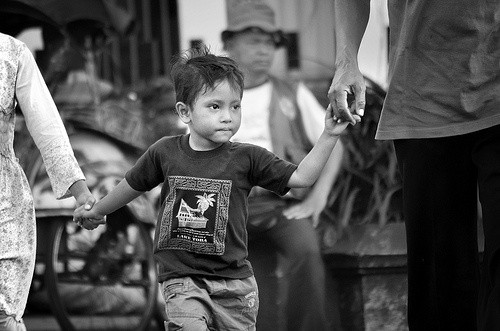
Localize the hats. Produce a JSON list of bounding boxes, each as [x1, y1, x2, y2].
[[221, 1, 282, 42]]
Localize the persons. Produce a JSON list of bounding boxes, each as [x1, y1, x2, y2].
[[73, 45, 355, 331], [326, 0, 500, 331], [0, 33, 107, 330], [185, 0, 346, 331]]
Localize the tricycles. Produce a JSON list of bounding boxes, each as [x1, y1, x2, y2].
[[19, 146, 160, 331]]
